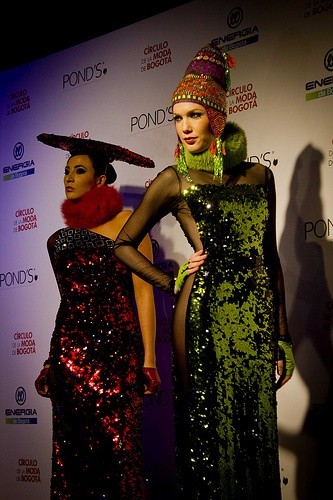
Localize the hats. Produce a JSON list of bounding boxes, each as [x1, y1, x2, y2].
[[37, 131, 155, 169], [171, 42, 234, 138]]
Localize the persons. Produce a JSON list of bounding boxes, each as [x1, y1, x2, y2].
[[109, 75, 295, 500], [34, 131, 162, 500]]
